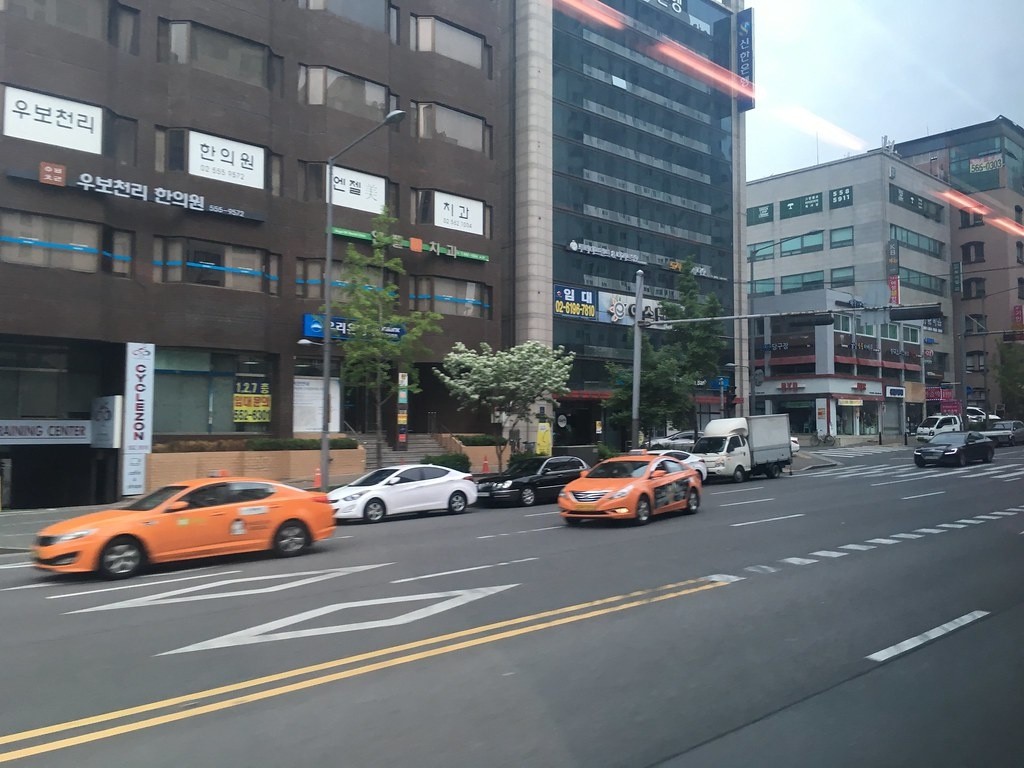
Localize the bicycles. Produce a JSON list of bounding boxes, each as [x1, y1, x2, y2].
[[809, 429, 835, 447]]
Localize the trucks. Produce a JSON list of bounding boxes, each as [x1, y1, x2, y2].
[[915, 415, 964, 443], [691, 414, 793, 484]]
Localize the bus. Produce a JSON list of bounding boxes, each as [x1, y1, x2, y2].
[[631, 448, 708, 484]]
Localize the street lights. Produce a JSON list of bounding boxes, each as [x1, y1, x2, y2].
[[724, 227, 825, 416], [295, 109, 407, 495]]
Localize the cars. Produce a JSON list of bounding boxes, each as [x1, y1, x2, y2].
[[644, 429, 706, 451], [557, 448, 703, 527], [328, 464, 478, 524], [965, 406, 1001, 422], [987, 420, 1024, 447], [30, 469, 337, 581], [477, 455, 591, 508], [913, 430, 994, 468], [791, 437, 800, 453]]
